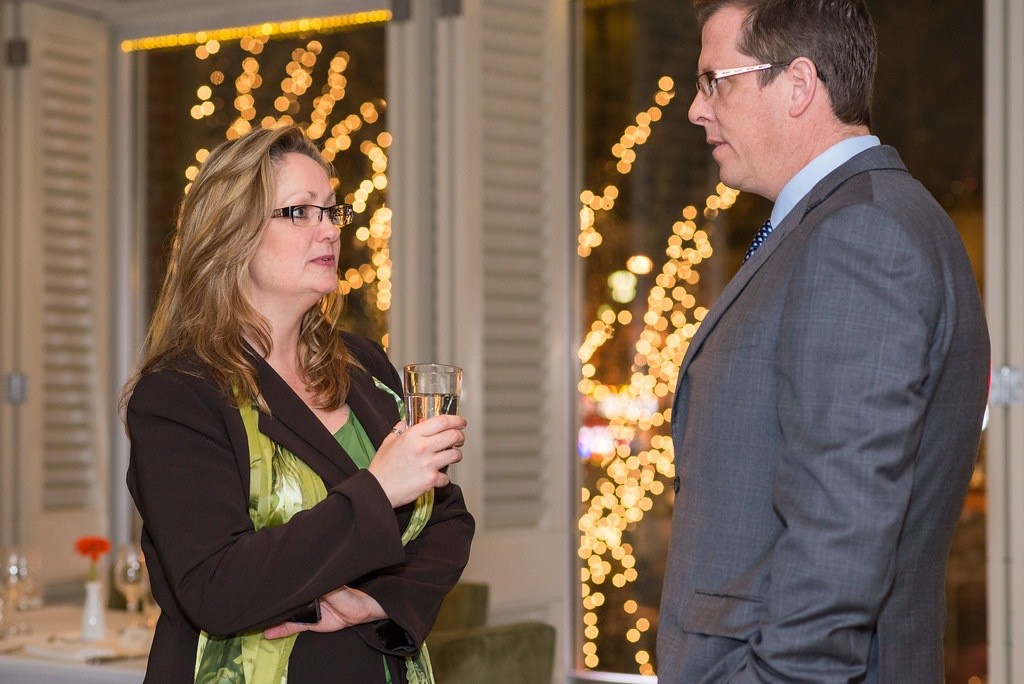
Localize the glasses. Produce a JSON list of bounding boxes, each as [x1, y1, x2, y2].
[[695, 58, 823, 101], [271, 204, 353, 227]]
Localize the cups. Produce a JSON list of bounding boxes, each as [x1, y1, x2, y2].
[[404, 364, 465, 472]]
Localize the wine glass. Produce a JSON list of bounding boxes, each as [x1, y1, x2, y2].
[[3, 544, 41, 637], [113, 542, 154, 648]]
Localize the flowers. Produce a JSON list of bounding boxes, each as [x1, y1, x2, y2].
[[74, 537, 112, 583]]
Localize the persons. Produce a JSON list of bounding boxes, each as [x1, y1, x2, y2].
[[655, 0, 993, 684], [112, 123, 477, 684]]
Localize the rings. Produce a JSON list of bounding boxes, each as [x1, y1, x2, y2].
[[390, 428, 402, 436]]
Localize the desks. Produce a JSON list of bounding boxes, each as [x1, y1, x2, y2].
[[0, 603, 163, 684]]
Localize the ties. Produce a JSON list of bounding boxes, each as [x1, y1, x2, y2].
[[742, 220, 772, 267]]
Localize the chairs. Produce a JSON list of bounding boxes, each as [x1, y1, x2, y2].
[[431, 581, 490, 630], [431, 621, 553, 684]]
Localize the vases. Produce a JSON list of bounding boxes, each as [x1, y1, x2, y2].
[[82, 583, 106, 643]]
